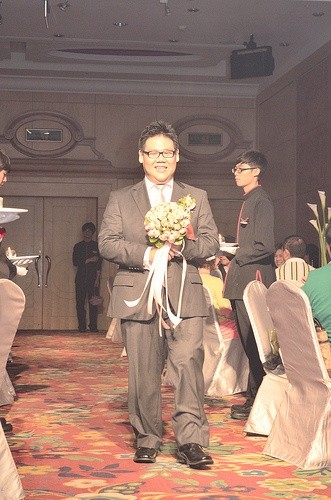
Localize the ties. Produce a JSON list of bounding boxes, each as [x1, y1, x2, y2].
[[153, 184, 167, 204]]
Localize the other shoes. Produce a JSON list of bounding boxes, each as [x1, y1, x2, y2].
[[232, 398, 252, 421]]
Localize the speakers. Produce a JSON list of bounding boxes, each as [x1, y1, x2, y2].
[[230, 45, 274, 79]]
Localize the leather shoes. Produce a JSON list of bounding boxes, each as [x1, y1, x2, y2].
[[132, 443, 160, 465], [172, 441, 214, 468]]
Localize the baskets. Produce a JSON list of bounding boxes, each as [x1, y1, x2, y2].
[[89, 285, 104, 306]]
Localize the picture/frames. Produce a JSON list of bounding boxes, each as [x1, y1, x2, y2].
[[26, 128, 63, 142], [186, 132, 223, 147]]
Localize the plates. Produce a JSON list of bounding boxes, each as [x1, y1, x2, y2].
[[0, 208, 28, 222], [220, 243, 239, 247], [8, 256, 39, 265]]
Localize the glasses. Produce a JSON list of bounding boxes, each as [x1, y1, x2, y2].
[[230, 167, 256, 173], [140, 147, 177, 159]]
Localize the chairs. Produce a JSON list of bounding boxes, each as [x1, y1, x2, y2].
[[106, 280, 117, 340], [242, 280, 288, 438], [0, 422, 26, 500], [0, 279, 25, 406], [201, 286, 250, 398], [262, 281, 331, 471]]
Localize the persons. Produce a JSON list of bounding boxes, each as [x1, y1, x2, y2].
[[0, 150, 28, 431], [98, 120, 220, 466], [196, 234, 331, 348], [72, 222, 102, 332], [220, 151, 275, 420]]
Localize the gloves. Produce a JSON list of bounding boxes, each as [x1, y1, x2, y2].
[[15, 263, 30, 275]]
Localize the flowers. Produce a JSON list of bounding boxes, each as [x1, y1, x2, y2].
[[89, 288, 104, 306], [124, 194, 197, 338]]
